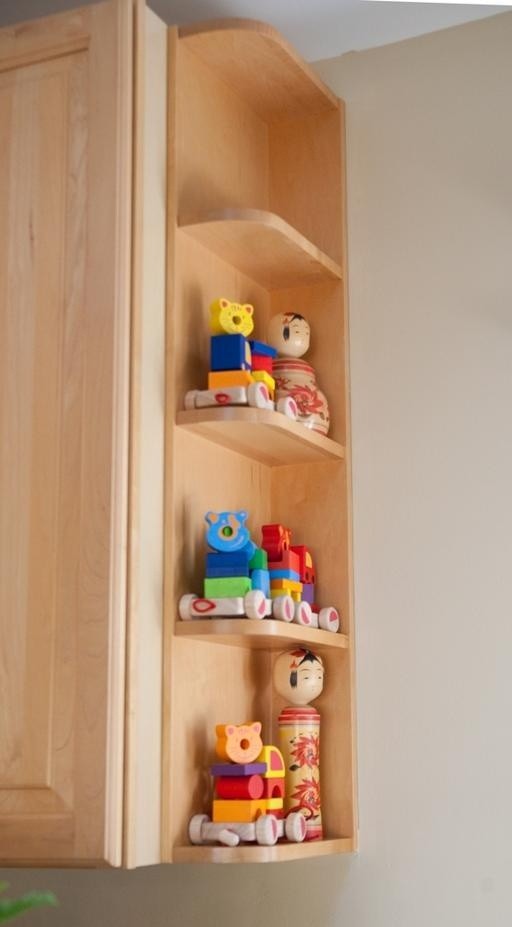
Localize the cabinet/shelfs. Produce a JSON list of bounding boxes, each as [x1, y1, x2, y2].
[[1, 2, 135, 869], [160, 16, 360, 864]]
